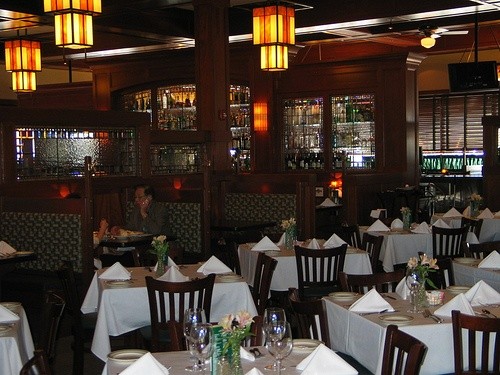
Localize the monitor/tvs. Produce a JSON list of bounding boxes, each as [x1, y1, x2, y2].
[[448, 60, 498, 95]]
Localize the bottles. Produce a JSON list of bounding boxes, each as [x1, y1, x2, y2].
[[162, 93, 197, 130], [286, 152, 343, 170], [232, 88, 250, 150]]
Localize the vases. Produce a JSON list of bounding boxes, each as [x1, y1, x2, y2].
[[157, 255, 166, 277], [231, 343, 243, 375]]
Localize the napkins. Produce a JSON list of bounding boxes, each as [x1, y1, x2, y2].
[[466, 280, 500, 306], [197, 255, 232, 275], [252, 236, 279, 253], [349, 289, 394, 313], [98, 262, 131, 281], [434, 293, 475, 317], [156, 266, 188, 282], [297, 344, 358, 375], [119, 352, 169, 375], [324, 233, 351, 249]]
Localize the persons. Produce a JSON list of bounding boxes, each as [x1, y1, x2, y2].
[[124, 183, 175, 270], [64, 192, 129, 270]]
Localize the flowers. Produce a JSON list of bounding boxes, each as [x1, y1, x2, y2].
[[408, 251, 440, 303], [279, 218, 296, 247], [223, 311, 255, 375], [151, 235, 169, 272]]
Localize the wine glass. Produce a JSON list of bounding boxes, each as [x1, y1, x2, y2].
[[263, 308, 292, 375], [183, 308, 214, 375]]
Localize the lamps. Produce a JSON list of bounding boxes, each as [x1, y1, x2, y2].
[[420, 27, 436, 48], [250, 2, 297, 71], [4, 0, 102, 91]]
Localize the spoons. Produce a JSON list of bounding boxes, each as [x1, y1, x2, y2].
[[422, 310, 443, 323]]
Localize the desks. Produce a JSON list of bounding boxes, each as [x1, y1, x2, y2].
[[0, 217, 500, 375], [235, 241, 373, 300], [350, 221, 482, 277], [81, 261, 258, 364], [102, 339, 358, 375], [432, 207, 500, 243], [322, 287, 500, 375], [0, 301, 39, 375], [451, 255, 500, 291]]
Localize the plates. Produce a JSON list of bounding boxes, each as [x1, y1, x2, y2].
[[104, 280, 134, 286], [216, 274, 246, 280], [114, 236, 128, 239], [0, 326, 8, 333], [330, 292, 357, 299], [446, 285, 473, 291], [287, 339, 325, 350], [0, 302, 20, 309], [454, 258, 477, 263], [13, 252, 33, 255], [107, 349, 150, 362], [378, 315, 414, 323]]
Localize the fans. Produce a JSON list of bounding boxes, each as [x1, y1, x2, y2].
[[394, 26, 469, 49]]
